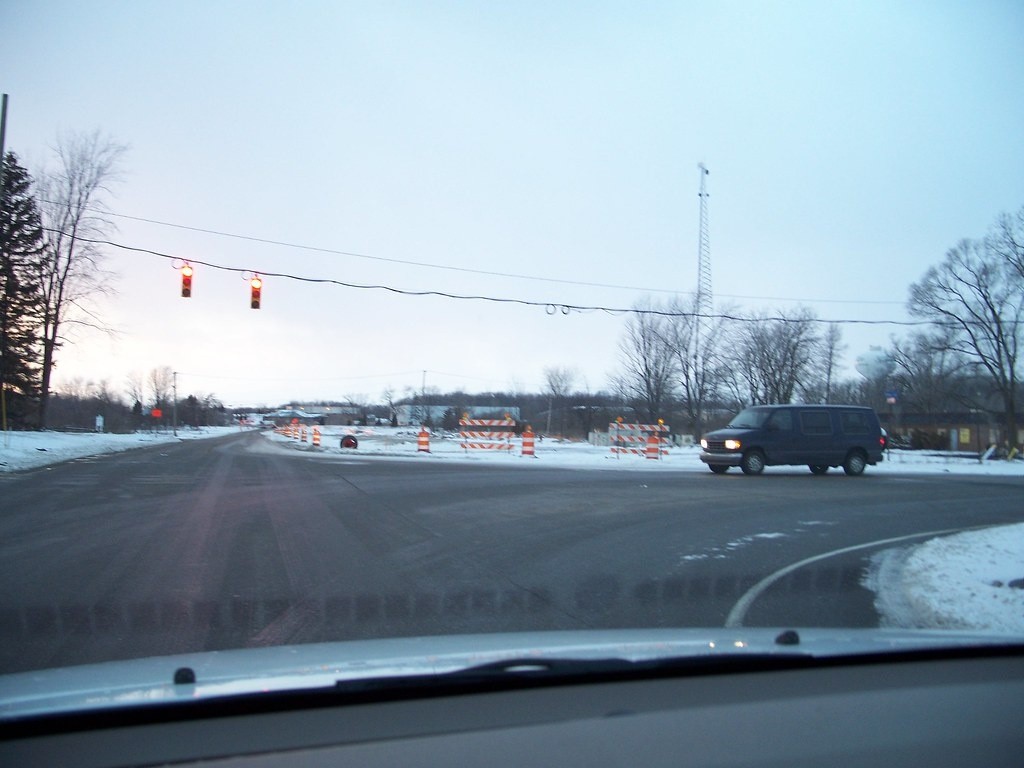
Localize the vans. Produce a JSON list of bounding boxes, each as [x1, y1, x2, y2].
[[699, 404, 888, 476]]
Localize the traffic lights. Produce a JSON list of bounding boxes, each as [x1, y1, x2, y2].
[[251, 277, 261, 309], [182, 266, 192, 297]]
